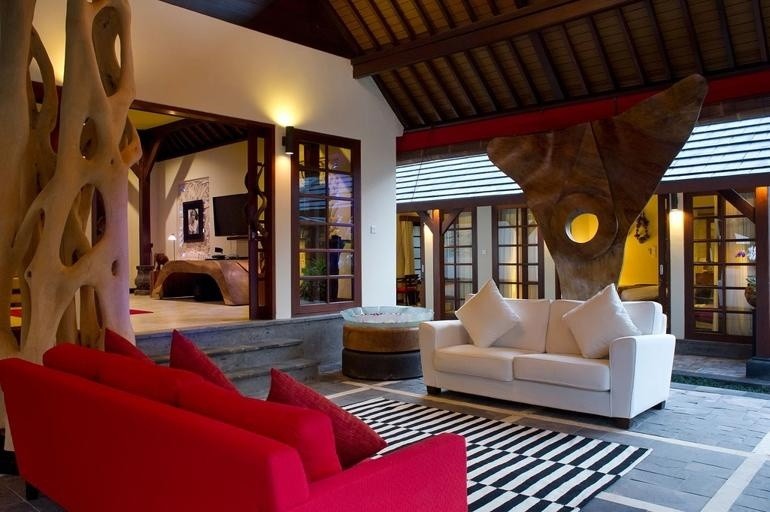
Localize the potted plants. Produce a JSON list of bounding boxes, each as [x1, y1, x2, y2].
[[745, 274, 756, 310], [299, 254, 328, 304]]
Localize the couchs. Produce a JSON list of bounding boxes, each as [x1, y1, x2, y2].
[[417, 291, 678, 430], [0, 341, 470, 512]]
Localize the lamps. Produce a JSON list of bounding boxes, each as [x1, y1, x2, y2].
[[281, 125, 296, 157], [167, 233, 178, 262], [670, 193, 679, 210]]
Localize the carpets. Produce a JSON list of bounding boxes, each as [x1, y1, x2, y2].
[[337, 395, 655, 512], [130, 307, 154, 317]]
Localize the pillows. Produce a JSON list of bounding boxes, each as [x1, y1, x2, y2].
[[167, 329, 239, 395], [264, 367, 387, 466], [454, 278, 522, 348], [562, 281, 641, 359], [103, 327, 152, 361]]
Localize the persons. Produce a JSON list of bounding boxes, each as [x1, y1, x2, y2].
[[187, 209, 199, 234]]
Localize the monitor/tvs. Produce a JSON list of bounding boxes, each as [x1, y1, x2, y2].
[[213, 193, 249, 236]]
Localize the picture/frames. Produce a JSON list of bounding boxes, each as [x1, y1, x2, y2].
[[182, 199, 205, 243]]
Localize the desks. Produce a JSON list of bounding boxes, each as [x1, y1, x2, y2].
[[153, 259, 249, 307]]
